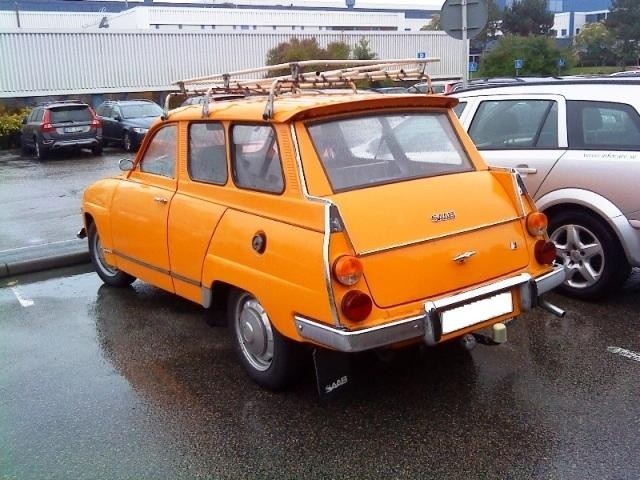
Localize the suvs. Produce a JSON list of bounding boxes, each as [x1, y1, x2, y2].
[[19, 101, 105, 160]]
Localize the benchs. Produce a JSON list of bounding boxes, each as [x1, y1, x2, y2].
[[328, 161, 393, 190]]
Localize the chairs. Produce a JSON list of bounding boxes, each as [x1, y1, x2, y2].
[[581, 106, 603, 144], [197, 143, 244, 182], [483, 111, 520, 148]]
